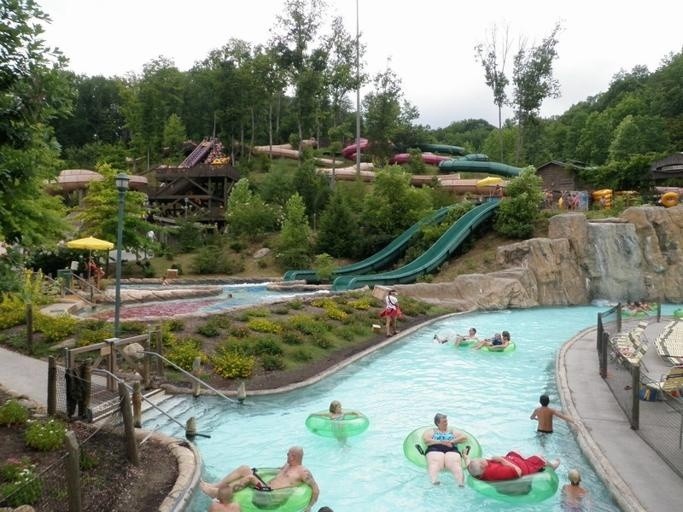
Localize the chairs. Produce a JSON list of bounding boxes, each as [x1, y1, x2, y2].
[[608, 315, 683, 401]]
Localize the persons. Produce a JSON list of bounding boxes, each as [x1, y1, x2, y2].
[[600, 195, 606, 209], [563, 470, 587, 512], [198, 446, 318, 512], [421, 413, 464, 487], [468, 450, 560, 482], [530, 394, 575, 432], [313, 401, 359, 419], [82, 253, 105, 283], [319, 507, 334, 512], [379, 289, 400, 336], [432, 328, 511, 351], [207, 487, 240, 512], [622, 297, 654, 318]]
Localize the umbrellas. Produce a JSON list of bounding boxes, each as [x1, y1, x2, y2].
[[479, 176, 503, 195], [65, 234, 114, 276]]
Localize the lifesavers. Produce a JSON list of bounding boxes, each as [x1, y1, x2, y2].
[[674, 309, 683, 319], [403, 426, 483, 470], [481, 340, 515, 353], [618, 303, 657, 317], [229, 468, 312, 512], [557, 188, 680, 211], [306, 409, 368, 437], [450, 339, 478, 348], [465, 465, 559, 503]]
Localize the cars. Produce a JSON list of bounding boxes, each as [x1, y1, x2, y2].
[[20, 247, 88, 281]]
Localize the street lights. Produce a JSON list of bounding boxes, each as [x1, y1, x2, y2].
[[114, 172, 129, 365]]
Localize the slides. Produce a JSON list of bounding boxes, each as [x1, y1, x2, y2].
[[283, 206, 448, 284], [331, 200, 501, 291]]
